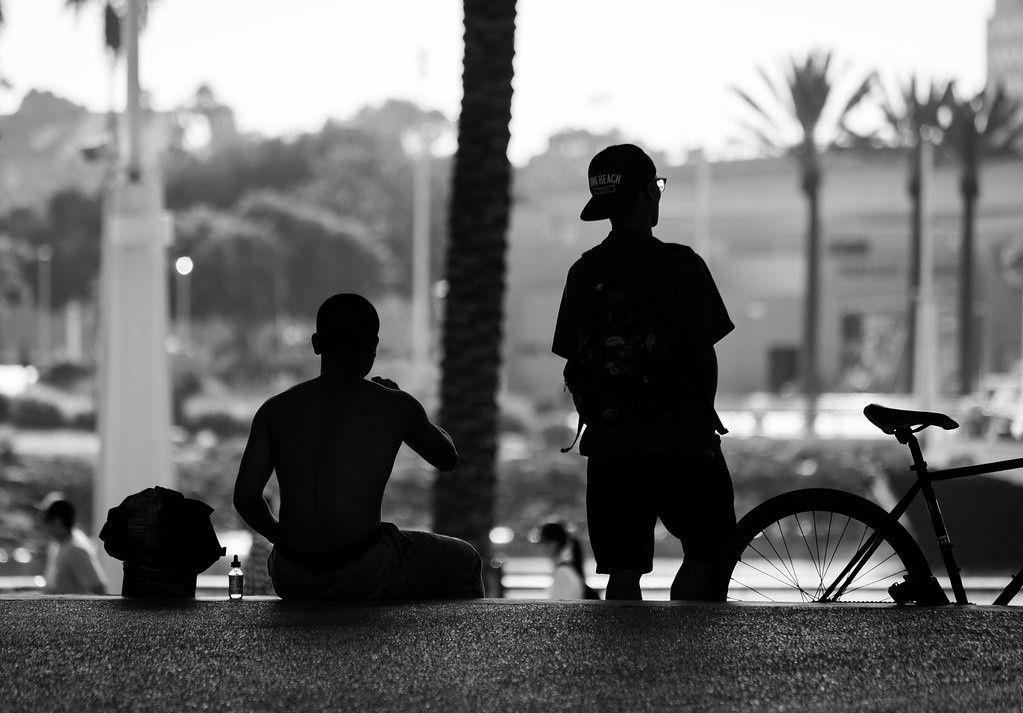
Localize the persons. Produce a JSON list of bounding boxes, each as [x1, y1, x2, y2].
[[39, 499, 104, 595], [549, 142, 737, 602], [539, 523, 590, 601], [240, 496, 278, 596], [234, 293, 488, 604]]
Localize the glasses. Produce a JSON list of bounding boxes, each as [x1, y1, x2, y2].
[[656, 178, 667, 193]]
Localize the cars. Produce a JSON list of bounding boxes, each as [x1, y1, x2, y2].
[[0, 348, 588, 584]]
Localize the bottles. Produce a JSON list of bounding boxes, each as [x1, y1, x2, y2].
[[228, 555, 244, 600]]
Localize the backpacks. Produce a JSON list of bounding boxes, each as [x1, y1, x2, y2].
[[559, 562, 605, 600]]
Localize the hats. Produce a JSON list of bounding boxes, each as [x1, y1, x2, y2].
[[580, 144, 657, 222], [30, 500, 76, 520], [527, 523, 574, 543]]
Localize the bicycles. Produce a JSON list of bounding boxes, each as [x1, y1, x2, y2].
[[721, 403, 1023, 606]]
